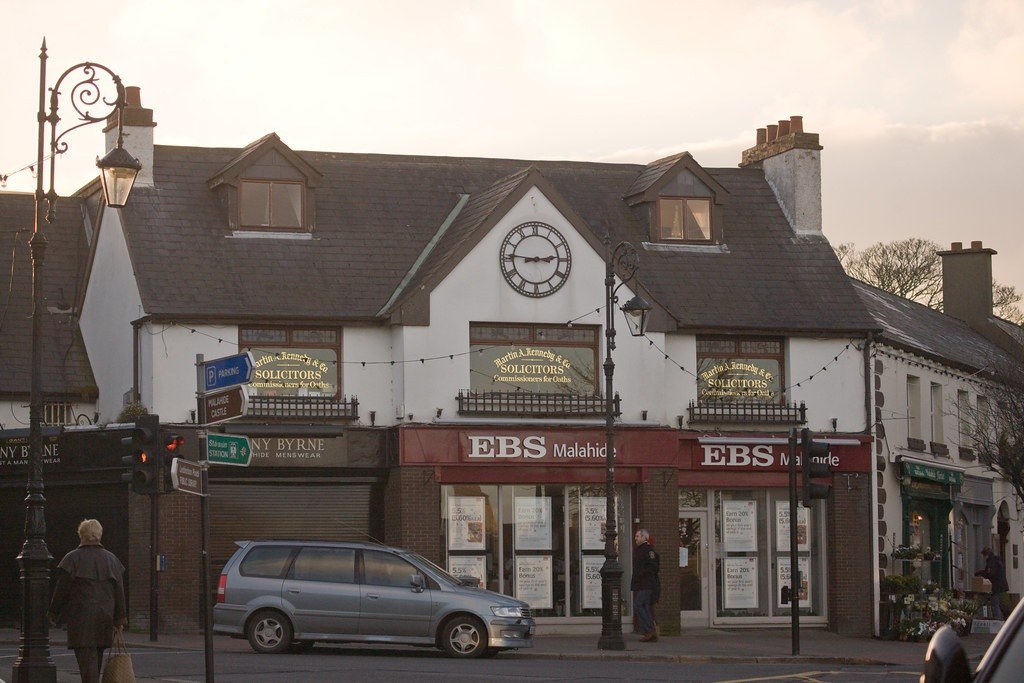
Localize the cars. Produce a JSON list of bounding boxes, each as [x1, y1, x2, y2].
[[919, 595, 1024, 683]]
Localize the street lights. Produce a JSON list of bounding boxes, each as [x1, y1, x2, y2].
[[592, 225, 654, 649], [9, 34, 140, 683]]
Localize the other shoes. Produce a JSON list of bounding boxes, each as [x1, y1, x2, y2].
[[640, 630, 658, 643], [984, 616, 1003, 621]]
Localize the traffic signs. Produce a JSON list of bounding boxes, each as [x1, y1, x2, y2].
[[206, 433, 252, 466], [205, 382, 249, 425], [171, 458, 204, 496], [202, 351, 256, 393]]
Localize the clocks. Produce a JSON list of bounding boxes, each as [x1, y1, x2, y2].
[[500, 222, 573, 298]]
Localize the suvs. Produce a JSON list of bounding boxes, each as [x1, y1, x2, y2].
[[208, 537, 537, 660]]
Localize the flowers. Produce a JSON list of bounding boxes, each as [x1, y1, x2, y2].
[[898, 619, 912, 635], [907, 545, 920, 554], [895, 547, 908, 556], [925, 547, 934, 555]]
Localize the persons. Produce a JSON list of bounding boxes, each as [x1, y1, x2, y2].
[[49, 519, 129, 683], [975, 547, 1008, 620], [630, 531, 660, 643]]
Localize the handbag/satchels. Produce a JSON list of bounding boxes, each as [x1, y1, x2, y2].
[[102, 630, 136, 683]]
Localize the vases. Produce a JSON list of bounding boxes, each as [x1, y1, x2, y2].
[[896, 554, 905, 559], [924, 553, 934, 560], [906, 554, 918, 560], [899, 633, 908, 641]]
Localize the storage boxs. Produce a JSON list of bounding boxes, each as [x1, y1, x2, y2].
[[972, 577, 992, 592], [971, 620, 1004, 634]]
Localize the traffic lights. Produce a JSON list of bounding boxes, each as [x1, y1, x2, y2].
[[159, 429, 187, 491], [130, 414, 160, 497], [121, 437, 134, 487], [801, 425, 836, 507]]
